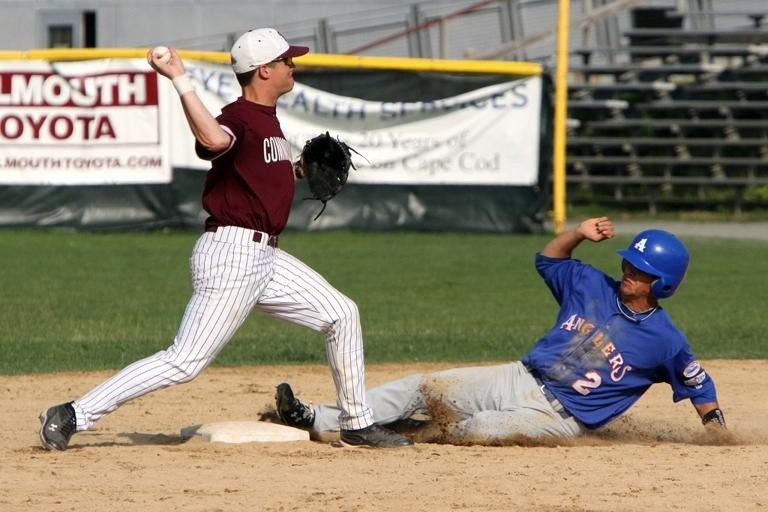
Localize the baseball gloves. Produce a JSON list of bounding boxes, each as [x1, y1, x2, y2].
[[298, 131, 371, 220]]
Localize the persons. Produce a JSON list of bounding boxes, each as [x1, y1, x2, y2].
[[38, 26, 416, 449], [275, 217, 742, 447]]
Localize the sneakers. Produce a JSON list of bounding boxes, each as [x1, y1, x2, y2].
[[40, 401, 76, 451], [340, 423, 413, 447], [275, 383, 314, 425]]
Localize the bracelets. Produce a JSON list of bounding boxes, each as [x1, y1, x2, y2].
[[171, 73, 195, 98], [701, 407, 727, 431]]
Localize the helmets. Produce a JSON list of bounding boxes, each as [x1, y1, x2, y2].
[[617, 229, 689, 298]]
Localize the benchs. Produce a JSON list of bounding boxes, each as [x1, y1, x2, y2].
[[546, 28, 768, 216]]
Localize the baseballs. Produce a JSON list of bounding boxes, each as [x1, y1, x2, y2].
[[153, 47, 170, 64]]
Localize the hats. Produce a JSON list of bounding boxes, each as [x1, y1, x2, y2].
[[231, 27, 309, 75]]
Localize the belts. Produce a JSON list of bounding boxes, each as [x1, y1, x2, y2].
[[206, 225, 278, 247], [522, 361, 572, 419]]
